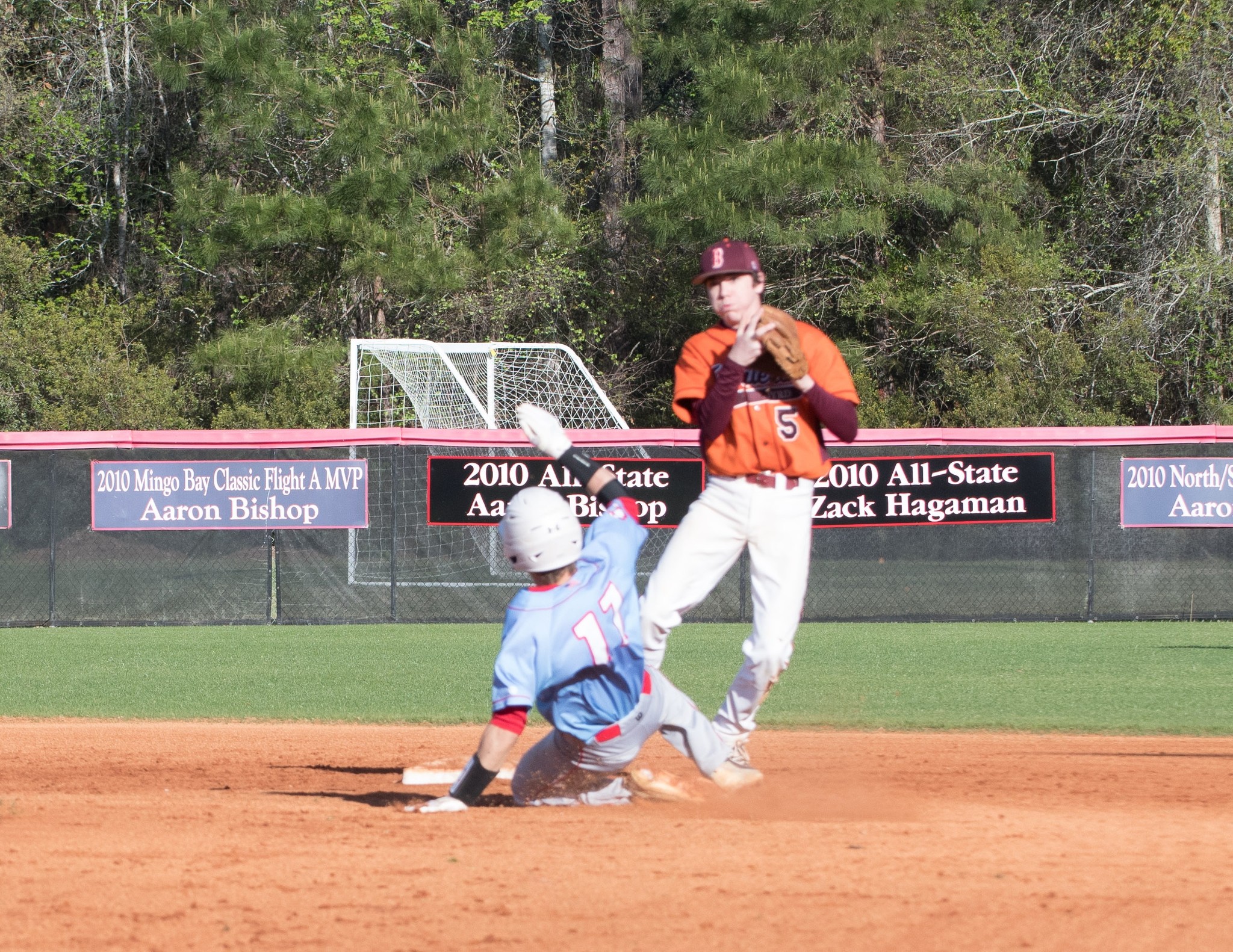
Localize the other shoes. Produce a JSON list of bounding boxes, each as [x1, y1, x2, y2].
[[712, 761, 762, 791], [730, 741, 749, 766], [623, 768, 705, 804]]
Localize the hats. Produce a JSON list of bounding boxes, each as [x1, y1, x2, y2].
[[692, 238, 761, 285]]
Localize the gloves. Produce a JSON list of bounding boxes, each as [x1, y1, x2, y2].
[[404, 796, 468, 813], [516, 403, 573, 460]]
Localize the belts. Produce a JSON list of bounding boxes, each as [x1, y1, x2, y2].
[[562, 671, 651, 746], [746, 473, 799, 490]]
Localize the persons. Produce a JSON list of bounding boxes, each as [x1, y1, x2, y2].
[[639, 237, 860, 767], [403, 403, 765, 813]]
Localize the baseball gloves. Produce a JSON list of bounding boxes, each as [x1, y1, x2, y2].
[[746, 305, 809, 381]]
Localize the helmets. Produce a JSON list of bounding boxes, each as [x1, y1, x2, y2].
[[501, 486, 583, 572]]
